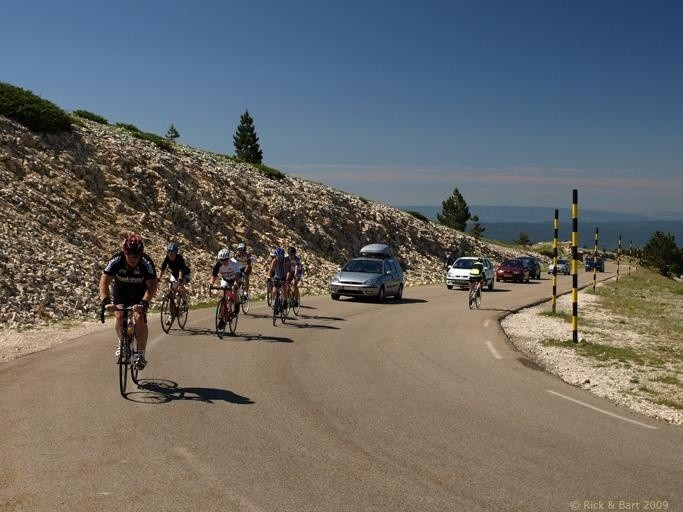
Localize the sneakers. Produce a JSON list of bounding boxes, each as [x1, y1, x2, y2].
[[134, 355, 146, 370], [116, 344, 128, 357]]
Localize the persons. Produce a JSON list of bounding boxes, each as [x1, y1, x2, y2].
[[157, 242, 302, 329], [99, 236, 157, 370], [469, 259, 487, 305]]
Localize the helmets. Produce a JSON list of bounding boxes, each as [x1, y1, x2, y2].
[[271, 246, 296, 258], [166, 242, 178, 253], [122, 236, 143, 255], [237, 242, 246, 250], [218, 248, 230, 261]]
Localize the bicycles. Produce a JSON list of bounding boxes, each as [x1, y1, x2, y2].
[[99, 302, 152, 396], [157, 277, 190, 335], [230, 277, 250, 316], [204, 284, 241, 340], [468, 278, 482, 309], [263, 275, 300, 327]]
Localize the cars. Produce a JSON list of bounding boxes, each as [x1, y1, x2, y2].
[[495, 259, 529, 283], [517, 256, 542, 282], [546, 258, 570, 275], [328, 244, 403, 301], [444, 256, 494, 291], [584, 257, 605, 273]]
